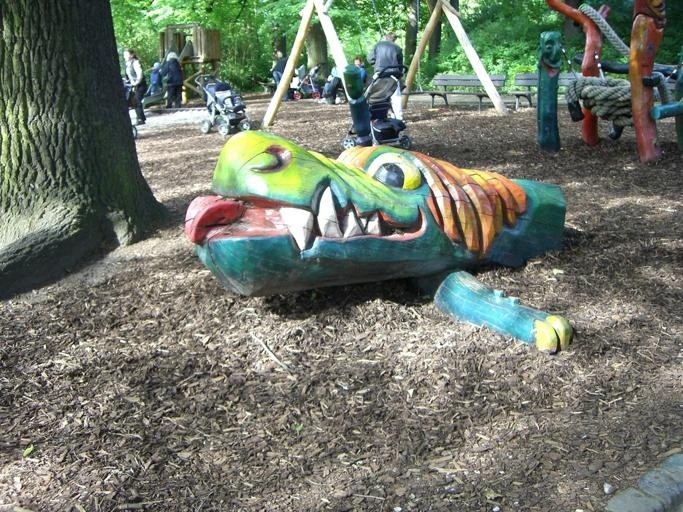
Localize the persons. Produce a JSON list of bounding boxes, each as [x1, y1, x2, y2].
[[321, 56, 367, 99], [272, 56, 296, 103], [318, 66, 341, 104], [123, 48, 148, 127], [366, 32, 406, 124], [268, 50, 284, 74], [158, 50, 182, 108], [149, 61, 161, 96]]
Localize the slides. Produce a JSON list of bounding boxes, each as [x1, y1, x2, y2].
[[141, 40, 194, 108]]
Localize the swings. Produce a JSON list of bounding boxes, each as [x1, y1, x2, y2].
[[350, 0, 424, 95]]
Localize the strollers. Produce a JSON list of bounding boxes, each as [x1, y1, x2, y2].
[[339, 64, 413, 150], [293, 61, 328, 100], [194, 73, 252, 136]]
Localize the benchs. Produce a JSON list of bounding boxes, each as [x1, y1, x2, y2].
[[426, 73, 580, 113]]
